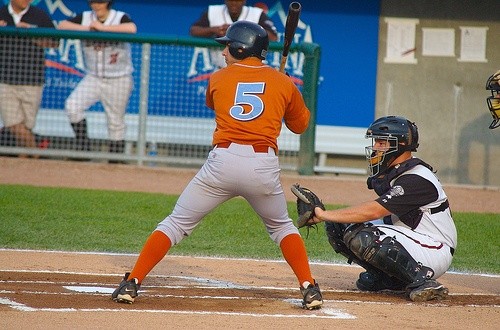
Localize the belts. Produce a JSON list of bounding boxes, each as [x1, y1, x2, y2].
[[217, 141, 268, 152], [450, 248, 454, 256]]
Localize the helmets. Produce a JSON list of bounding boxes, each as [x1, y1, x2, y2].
[[486, 72, 500, 129], [365, 116, 419, 177], [89, 0, 112, 6], [215, 21, 269, 60]]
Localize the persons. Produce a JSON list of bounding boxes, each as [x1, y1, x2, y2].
[[0, 0, 61, 159], [292, 116, 458, 301], [112, 21, 323, 310], [190, 0, 278, 75], [57, 0, 137, 164], [486, 71, 500, 129]]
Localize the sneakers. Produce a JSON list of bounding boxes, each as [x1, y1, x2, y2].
[[356, 272, 406, 292], [300, 279, 323, 310], [112, 272, 140, 305], [410, 279, 449, 301]]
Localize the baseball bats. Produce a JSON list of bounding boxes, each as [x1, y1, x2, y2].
[[278, 1, 302, 71]]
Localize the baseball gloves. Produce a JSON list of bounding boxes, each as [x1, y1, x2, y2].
[[288, 184, 326, 230]]
